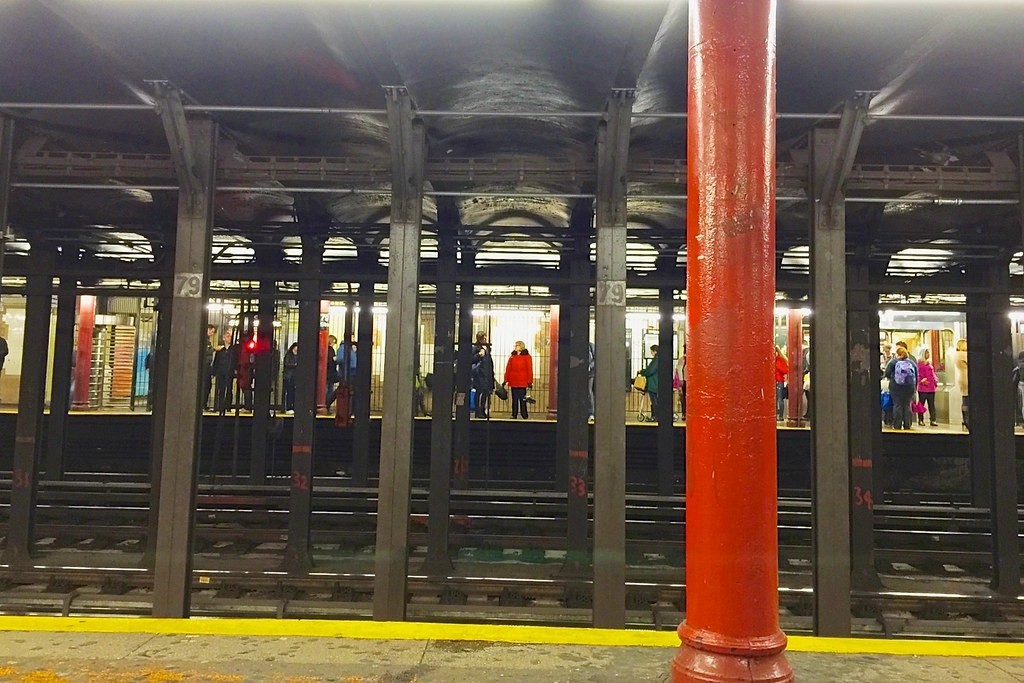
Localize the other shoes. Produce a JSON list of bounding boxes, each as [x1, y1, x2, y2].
[[893, 426, 901, 429], [918, 420, 926, 426], [903, 424, 910, 429], [509, 415, 517, 419], [522, 415, 528, 419], [145, 399, 338, 417], [681, 415, 686, 421], [588, 415, 595, 422], [424, 413, 432, 417], [930, 421, 938, 426]]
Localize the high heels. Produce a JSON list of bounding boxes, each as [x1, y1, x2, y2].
[[475, 410, 485, 419], [962, 421, 968, 432], [481, 410, 492, 418]]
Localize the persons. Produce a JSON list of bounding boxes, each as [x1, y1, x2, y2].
[[677, 343, 687, 421], [1013, 350, 1024, 424], [775, 344, 788, 420], [203, 324, 298, 415], [0, 337, 8, 372], [416, 330, 534, 421], [802, 340, 812, 421], [879, 338, 969, 432], [326, 332, 358, 424], [637, 344, 658, 421], [588, 342, 595, 424]]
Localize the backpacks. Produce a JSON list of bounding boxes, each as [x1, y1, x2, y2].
[[892, 359, 916, 386]]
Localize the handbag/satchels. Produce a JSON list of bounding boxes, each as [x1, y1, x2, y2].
[[911, 400, 927, 413], [633, 371, 648, 393], [673, 371, 680, 388], [524, 387, 536, 405], [495, 382, 510, 400]]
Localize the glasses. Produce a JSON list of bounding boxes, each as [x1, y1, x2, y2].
[[514, 345, 522, 347]]
